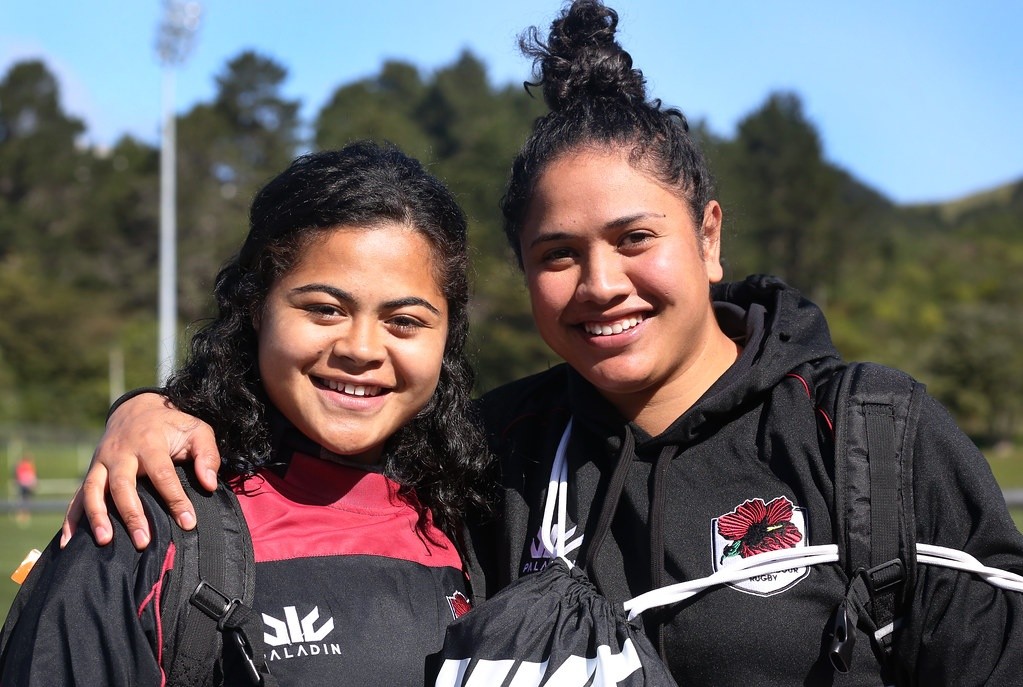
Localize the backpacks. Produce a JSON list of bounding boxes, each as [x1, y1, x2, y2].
[[432, 412, 1023, 687]]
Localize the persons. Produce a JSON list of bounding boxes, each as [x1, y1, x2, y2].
[[0, 0, 1023, 687]]
